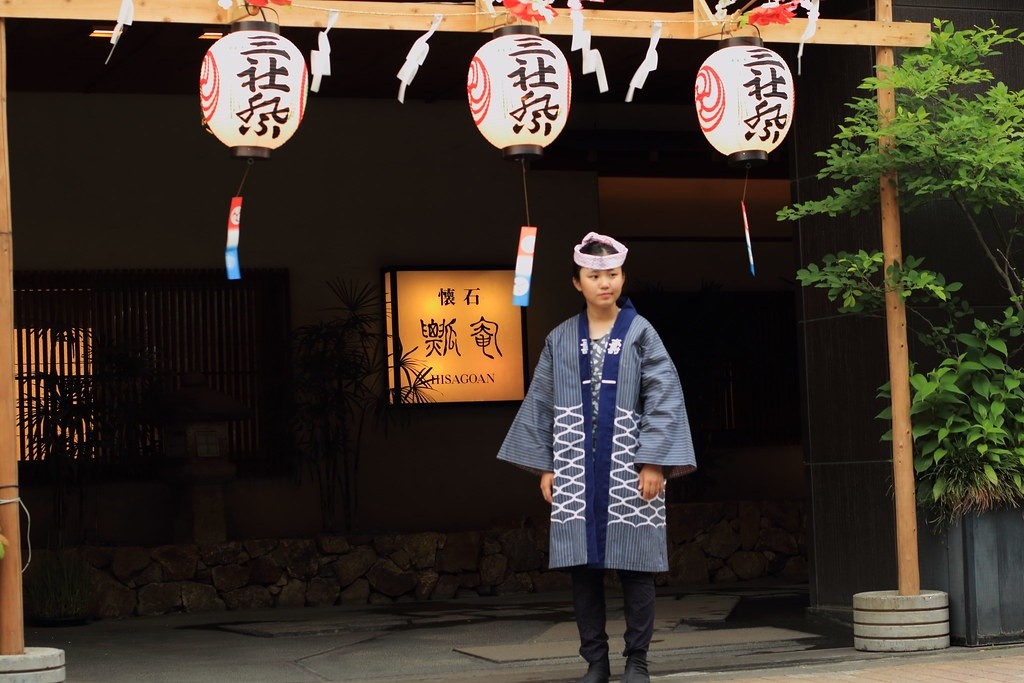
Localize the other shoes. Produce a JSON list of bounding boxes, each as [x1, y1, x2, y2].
[[578, 659, 611, 683], [619, 649, 650, 683]]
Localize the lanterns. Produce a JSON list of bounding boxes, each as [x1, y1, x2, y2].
[[198, 20, 310, 159], [466, 23, 573, 156], [693, 36, 798, 163]]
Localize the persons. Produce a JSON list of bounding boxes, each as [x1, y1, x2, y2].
[[495, 231, 697, 683]]
